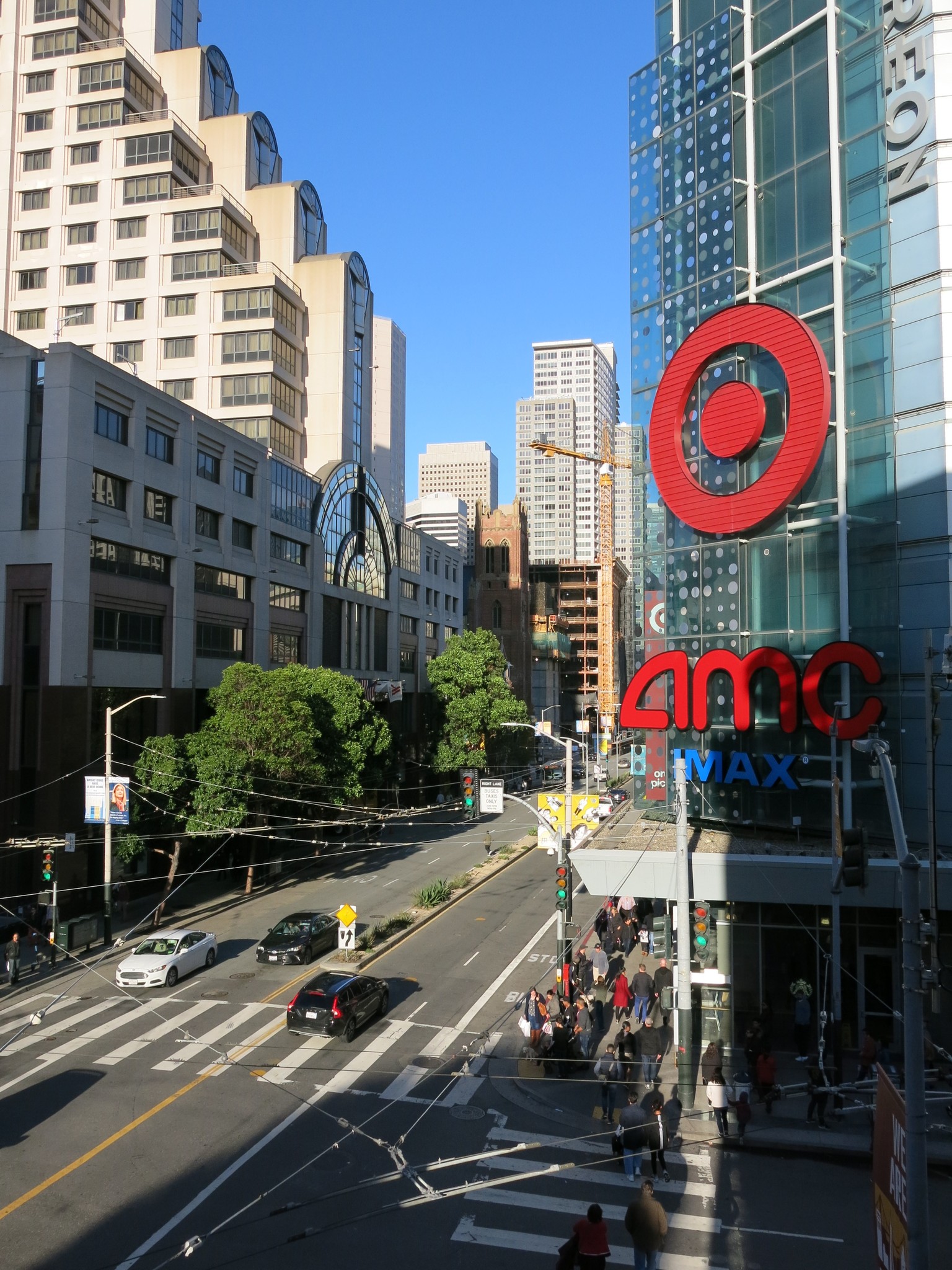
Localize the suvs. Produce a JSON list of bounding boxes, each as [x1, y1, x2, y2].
[[598, 788, 630, 819], [572, 763, 586, 778], [286, 971, 389, 1044]]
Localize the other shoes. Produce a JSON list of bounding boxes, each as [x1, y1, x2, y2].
[[718, 1131, 744, 1144], [608, 1119, 615, 1124], [645, 1084, 651, 1089], [596, 1028, 605, 1032], [601, 1114, 607, 1120], [642, 951, 648, 956], [616, 1016, 646, 1026], [651, 1076, 657, 1083], [556, 1074, 567, 1078], [625, 954, 628, 958], [623, 1083, 629, 1090]]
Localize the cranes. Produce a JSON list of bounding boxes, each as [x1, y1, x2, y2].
[[531, 422, 630, 730]]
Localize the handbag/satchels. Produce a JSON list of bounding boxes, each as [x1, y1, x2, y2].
[[609, 978, 617, 992], [558, 1231, 579, 1264], [518, 1016, 531, 1037], [537, 993, 547, 1016], [615, 940, 625, 952], [598, 1074, 608, 1085], [542, 1019, 553, 1037]]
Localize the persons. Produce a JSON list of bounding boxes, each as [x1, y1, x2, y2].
[[520, 777, 532, 796], [3, 932, 21, 986], [446, 792, 453, 809], [619, 1091, 671, 1182], [112, 876, 130, 922], [436, 791, 444, 810], [283, 922, 299, 934], [854, 1027, 875, 1089], [922, 1019, 934, 1091], [701, 1018, 777, 1146], [484, 831, 493, 856], [594, 896, 654, 958], [572, 1204, 610, 1270], [808, 1050, 832, 1130], [525, 943, 673, 1125], [625, 1180, 668, 1270], [21, 899, 60, 948]]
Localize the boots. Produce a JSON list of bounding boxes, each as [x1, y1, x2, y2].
[[533, 1029, 540, 1047], [529, 1029, 536, 1046]]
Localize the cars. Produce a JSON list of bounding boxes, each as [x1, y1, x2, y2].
[[588, 753, 597, 761], [115, 929, 218, 988], [618, 758, 630, 768], [593, 768, 610, 782], [255, 912, 339, 965]]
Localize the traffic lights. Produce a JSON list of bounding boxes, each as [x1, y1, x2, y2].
[[661, 990, 673, 1009], [554, 864, 567, 910], [694, 902, 710, 962], [459, 767, 482, 821], [38, 892, 51, 905], [41, 849, 54, 882], [842, 829, 865, 888], [606, 754, 609, 762], [651, 915, 672, 959]]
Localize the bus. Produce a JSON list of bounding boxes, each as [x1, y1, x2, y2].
[[541, 759, 565, 787]]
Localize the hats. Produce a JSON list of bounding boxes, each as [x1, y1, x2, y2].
[[560, 997, 570, 1002], [642, 924, 648, 930], [580, 945, 588, 949], [594, 943, 601, 948]]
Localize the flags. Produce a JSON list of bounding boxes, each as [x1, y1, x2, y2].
[[374, 680, 391, 693], [387, 681, 402, 703], [360, 679, 376, 701]]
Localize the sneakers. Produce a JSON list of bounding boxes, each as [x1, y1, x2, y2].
[[627, 1170, 670, 1183]]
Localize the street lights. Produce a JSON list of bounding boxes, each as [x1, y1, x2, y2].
[[541, 705, 560, 764], [852, 737, 925, 1270], [830, 702, 849, 1110], [616, 734, 623, 782], [581, 702, 597, 763], [104, 694, 166, 945], [500, 722, 573, 1007]]
